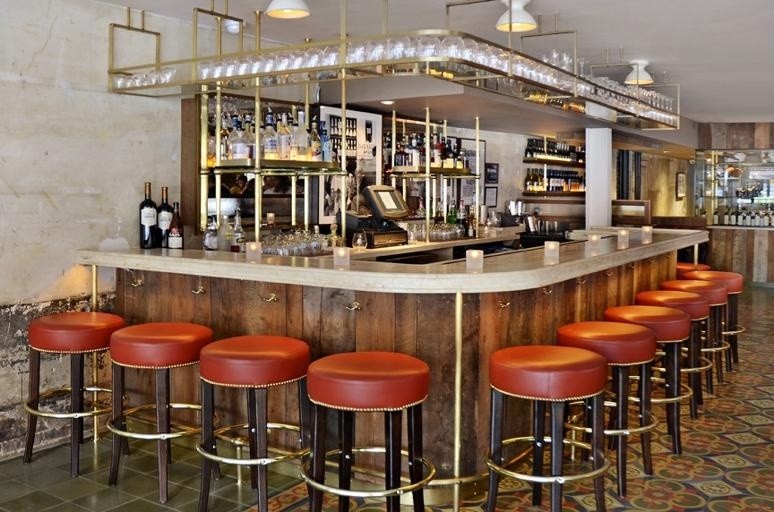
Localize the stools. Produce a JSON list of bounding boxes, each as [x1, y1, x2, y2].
[[193, 334, 312, 512], [108, 322, 223, 505], [487, 346, 605, 512], [605, 305, 692, 456], [641, 264, 743, 420], [18, 311, 129, 479], [297, 349, 437, 512], [558, 319, 653, 499]]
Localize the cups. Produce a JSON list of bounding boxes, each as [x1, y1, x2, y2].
[[406, 223, 464, 242], [331, 246, 349, 267], [246, 242, 260, 260], [352, 233, 367, 250], [588, 232, 602, 249], [489, 211, 501, 229], [617, 241, 628, 250], [465, 249, 483, 269], [553, 219, 559, 233], [641, 237, 652, 244], [112, 35, 673, 125], [544, 221, 550, 233], [258, 224, 342, 257], [544, 241, 559, 257], [617, 230, 629, 241], [641, 226, 653, 237], [266, 213, 274, 224]]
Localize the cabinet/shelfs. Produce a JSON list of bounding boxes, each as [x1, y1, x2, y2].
[[196, 88, 348, 249], [701, 162, 773, 201], [383, 113, 461, 226], [522, 156, 586, 198]]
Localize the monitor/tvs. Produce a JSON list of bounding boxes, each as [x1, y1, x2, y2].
[[361, 185, 410, 228]]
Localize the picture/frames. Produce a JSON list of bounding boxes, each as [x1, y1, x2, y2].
[[484, 187, 497, 207], [676, 172, 687, 197], [484, 163, 498, 184]]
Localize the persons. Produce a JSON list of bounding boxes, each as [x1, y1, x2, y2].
[[324, 146, 376, 215], [208, 175, 304, 198]]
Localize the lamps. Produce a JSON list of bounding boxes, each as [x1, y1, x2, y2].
[[623, 59, 653, 87], [494, 0, 537, 34], [265, 0, 312, 20]]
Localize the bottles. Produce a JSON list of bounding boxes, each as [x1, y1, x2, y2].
[[138, 181, 158, 248], [203, 109, 332, 164], [383, 129, 468, 169], [156, 185, 174, 247], [699, 207, 774, 228], [167, 202, 184, 249], [525, 138, 584, 162], [523, 168, 582, 191], [202, 208, 246, 252], [434, 199, 487, 236]]
